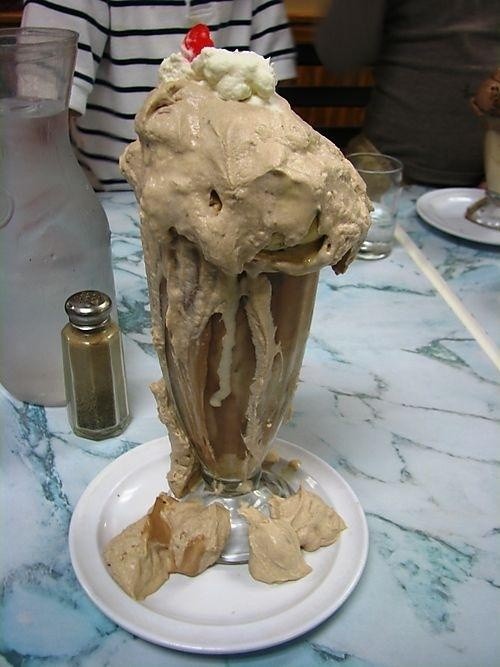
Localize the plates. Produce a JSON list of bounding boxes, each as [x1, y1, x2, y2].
[[67, 433, 370, 655], [416, 188, 500, 246]]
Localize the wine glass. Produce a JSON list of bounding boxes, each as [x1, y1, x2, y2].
[[146, 219, 335, 562]]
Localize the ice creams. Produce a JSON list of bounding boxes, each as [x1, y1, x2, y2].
[[103, 24, 373, 601], [465, 65, 500, 230]]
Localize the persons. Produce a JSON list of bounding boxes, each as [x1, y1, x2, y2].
[[312, 1, 500, 204], [14, 0, 299, 192]]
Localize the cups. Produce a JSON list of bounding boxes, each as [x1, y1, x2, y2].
[[344, 152, 403, 261]]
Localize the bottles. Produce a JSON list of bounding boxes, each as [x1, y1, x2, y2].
[[1, 24, 118, 409], [466, 115, 499, 229]]
